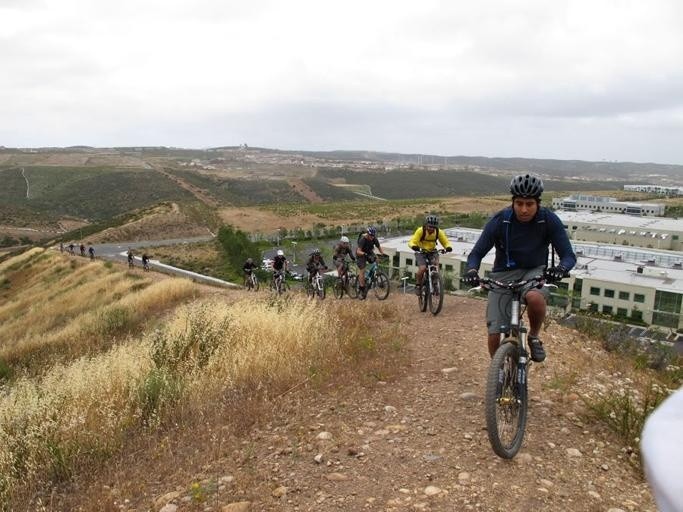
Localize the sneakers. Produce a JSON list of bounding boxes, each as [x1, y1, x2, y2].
[[496, 368, 505, 398], [415, 283, 422, 295], [527, 334, 546, 363]]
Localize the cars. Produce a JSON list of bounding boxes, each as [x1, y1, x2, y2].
[[397, 283, 409, 288], [260, 257, 306, 284]]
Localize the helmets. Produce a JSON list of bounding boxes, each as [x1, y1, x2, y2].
[[425, 215, 439, 227], [277, 249, 283, 256], [339, 236, 349, 243], [508, 173, 544, 200], [313, 248, 320, 256], [366, 226, 376, 236]]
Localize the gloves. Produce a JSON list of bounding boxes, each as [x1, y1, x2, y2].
[[544, 263, 568, 283], [411, 246, 420, 251], [445, 247, 452, 252], [461, 271, 480, 287]]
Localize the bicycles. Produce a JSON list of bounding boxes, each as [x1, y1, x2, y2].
[[330, 256, 363, 299], [68, 249, 151, 276], [454, 268, 570, 460], [353, 252, 393, 300], [305, 265, 327, 301], [413, 246, 452, 314], [241, 266, 261, 293], [269, 272, 291, 299]]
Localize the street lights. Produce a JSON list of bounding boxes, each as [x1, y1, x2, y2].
[[292, 241, 297, 264], [276, 228, 280, 247], [401, 275, 408, 295]]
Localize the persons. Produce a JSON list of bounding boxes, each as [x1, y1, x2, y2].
[[408, 215, 452, 296], [355, 227, 383, 298], [242, 258, 258, 288], [332, 236, 355, 293], [59, 240, 150, 273], [306, 249, 328, 285], [272, 250, 289, 283], [460, 174, 577, 395]]
